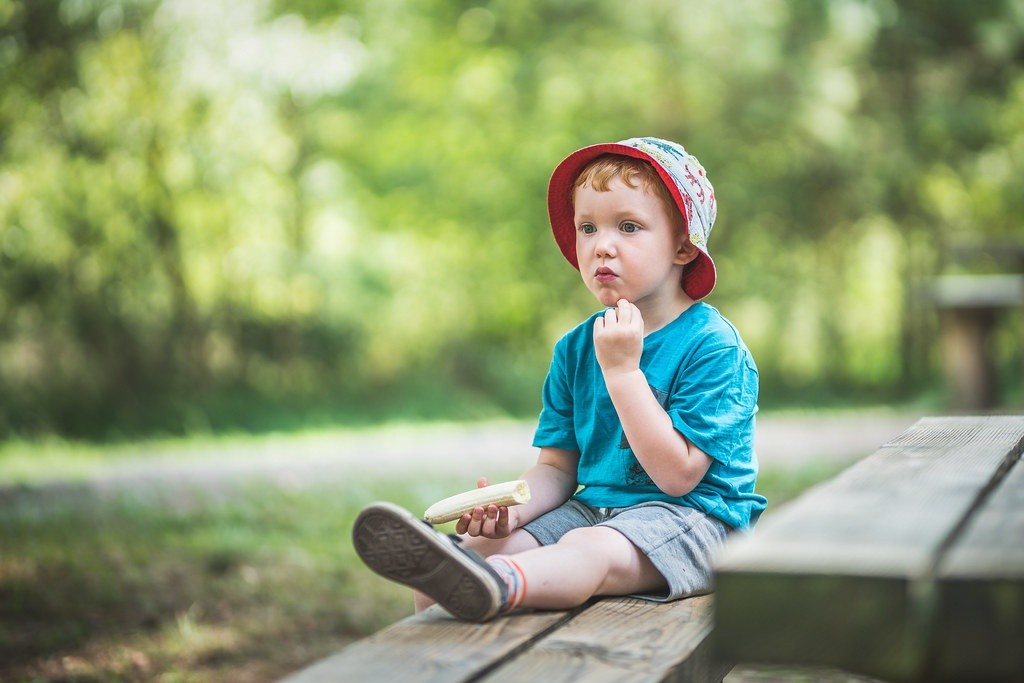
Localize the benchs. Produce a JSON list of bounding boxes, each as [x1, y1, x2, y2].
[[280, 592, 737, 683]]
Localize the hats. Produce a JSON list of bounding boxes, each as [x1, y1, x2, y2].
[[548, 136, 717, 301]]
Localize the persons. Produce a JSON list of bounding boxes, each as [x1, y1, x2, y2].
[[352, 138, 769, 622]]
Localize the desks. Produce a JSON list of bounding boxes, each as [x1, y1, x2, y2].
[[709, 416, 1024, 683], [932, 275, 1024, 414]]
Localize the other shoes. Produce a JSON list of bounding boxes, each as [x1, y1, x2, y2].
[[351, 501, 510, 623]]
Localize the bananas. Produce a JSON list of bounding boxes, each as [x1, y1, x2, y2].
[[424, 480, 531, 523]]
